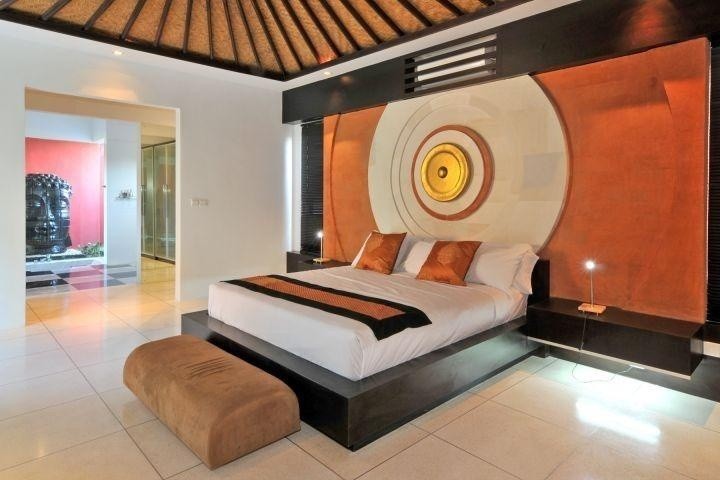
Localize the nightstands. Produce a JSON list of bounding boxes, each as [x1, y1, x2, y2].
[[297, 260, 351, 272], [528, 298, 704, 381]]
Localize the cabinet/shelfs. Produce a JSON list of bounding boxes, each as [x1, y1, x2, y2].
[[141, 147, 154, 256], [153, 142, 175, 263]]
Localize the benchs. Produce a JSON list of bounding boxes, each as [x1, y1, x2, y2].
[[123, 335, 301, 471]]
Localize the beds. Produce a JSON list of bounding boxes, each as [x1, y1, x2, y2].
[[182, 258, 550, 452]]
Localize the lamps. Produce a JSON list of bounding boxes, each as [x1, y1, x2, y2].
[[577, 260, 607, 314], [312, 230, 331, 264]]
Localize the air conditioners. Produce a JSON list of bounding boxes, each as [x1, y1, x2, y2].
[[414, 34, 496, 91]]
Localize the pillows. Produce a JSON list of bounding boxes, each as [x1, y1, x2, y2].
[[355, 231, 406, 275], [398, 239, 434, 276], [464, 243, 540, 295], [351, 230, 412, 271], [464, 248, 528, 296], [414, 240, 482, 286]]
